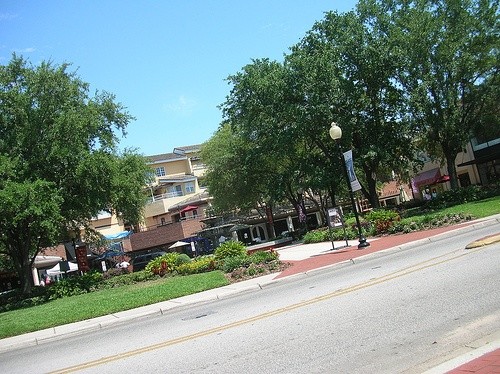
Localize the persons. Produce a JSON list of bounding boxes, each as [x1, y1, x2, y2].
[[120, 258, 131, 273], [243, 232, 249, 246], [432, 189, 436, 198], [425, 191, 431, 200], [115, 261, 120, 268]]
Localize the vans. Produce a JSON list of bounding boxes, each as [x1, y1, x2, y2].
[[127, 252, 167, 271]]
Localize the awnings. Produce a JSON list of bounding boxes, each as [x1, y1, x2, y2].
[[413, 167, 441, 187]]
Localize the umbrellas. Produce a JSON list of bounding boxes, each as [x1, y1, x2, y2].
[[168, 241, 191, 253], [180, 205, 198, 217]]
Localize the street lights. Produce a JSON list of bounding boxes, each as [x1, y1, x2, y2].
[[329, 121, 371, 250]]
